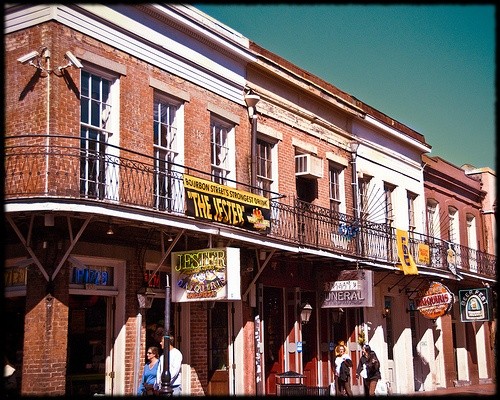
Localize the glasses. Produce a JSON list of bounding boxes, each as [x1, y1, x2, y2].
[[147, 351, 153, 354]]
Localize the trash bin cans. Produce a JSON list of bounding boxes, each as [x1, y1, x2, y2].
[[276, 370, 307, 396]]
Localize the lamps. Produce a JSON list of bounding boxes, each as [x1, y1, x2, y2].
[[297, 301, 313, 326], [136, 282, 155, 309], [106, 225, 114, 234], [329, 307, 345, 323], [44, 214, 55, 226]]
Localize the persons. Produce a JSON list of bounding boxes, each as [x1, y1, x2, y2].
[[356, 344, 381, 396], [334, 333, 363, 396], [137, 346, 161, 395], [153, 333, 183, 395]]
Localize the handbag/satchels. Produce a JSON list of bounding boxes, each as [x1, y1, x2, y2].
[[330, 372, 336, 396]]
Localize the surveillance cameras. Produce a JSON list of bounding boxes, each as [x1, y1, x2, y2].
[[17, 51, 43, 71], [58, 50, 84, 71]]
